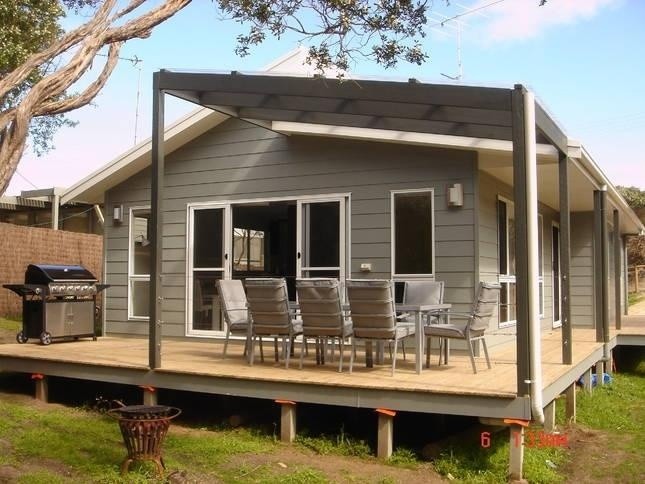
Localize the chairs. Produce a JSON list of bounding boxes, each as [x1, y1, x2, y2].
[[424, 281, 503, 377], [403, 278, 446, 367], [293, 278, 357, 373], [215, 276, 265, 362], [345, 278, 416, 377], [241, 275, 308, 373]]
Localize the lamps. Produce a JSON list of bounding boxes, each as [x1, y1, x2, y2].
[[137, 234, 150, 246]]
